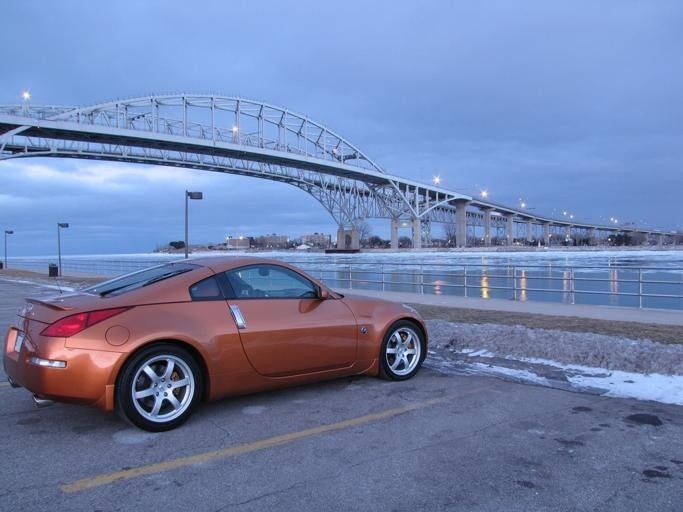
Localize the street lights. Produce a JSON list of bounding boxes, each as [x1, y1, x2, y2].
[[184, 189, 204, 258], [3, 228, 13, 268], [56, 220, 70, 275]]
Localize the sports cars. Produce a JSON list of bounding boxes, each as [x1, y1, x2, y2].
[[4, 251, 436, 430]]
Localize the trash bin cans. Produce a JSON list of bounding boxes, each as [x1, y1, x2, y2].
[[49, 263, 58, 277]]
